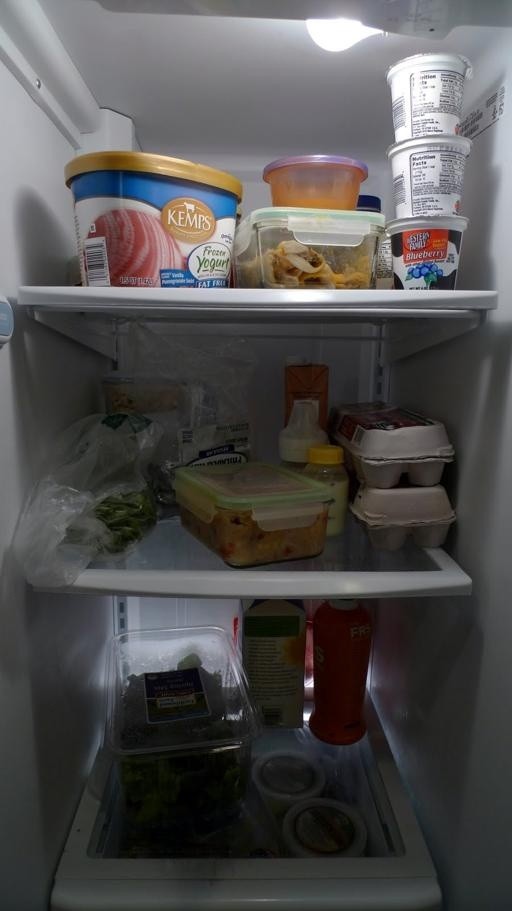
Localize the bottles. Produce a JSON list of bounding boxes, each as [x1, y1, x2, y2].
[[277, 399, 351, 543], [308, 597, 372, 744]]
